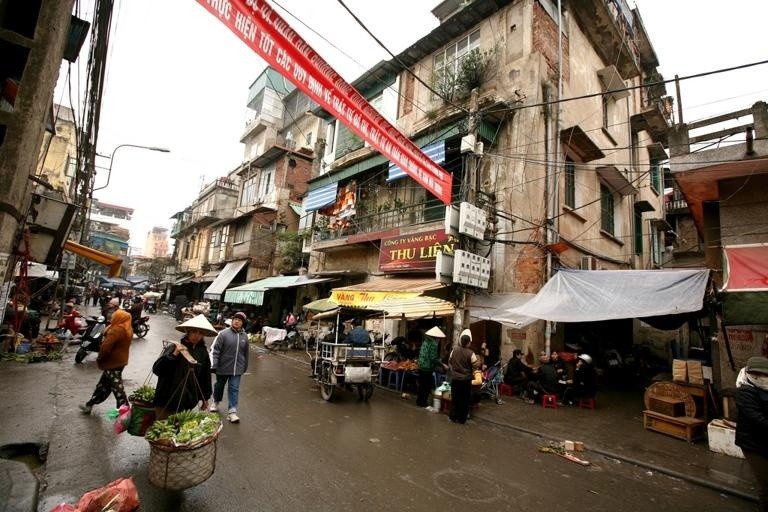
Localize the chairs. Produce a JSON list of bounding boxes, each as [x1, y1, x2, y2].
[[334, 346, 373, 363]]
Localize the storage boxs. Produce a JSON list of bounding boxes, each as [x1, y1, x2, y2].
[[649, 395, 686, 417], [707, 418, 745, 459]]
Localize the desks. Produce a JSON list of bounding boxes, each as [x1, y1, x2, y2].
[[652, 371, 713, 432]]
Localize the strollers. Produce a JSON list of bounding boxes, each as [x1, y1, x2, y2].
[[481, 360, 507, 405]]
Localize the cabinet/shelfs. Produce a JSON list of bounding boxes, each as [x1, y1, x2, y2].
[[642, 409, 706, 446]]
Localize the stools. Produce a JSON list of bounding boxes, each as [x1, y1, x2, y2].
[[543, 395, 557, 409], [499, 382, 512, 399], [579, 396, 597, 413], [387, 370, 400, 390], [378, 367, 389, 385]]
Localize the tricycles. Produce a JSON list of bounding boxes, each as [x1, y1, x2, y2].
[[305, 306, 388, 401]]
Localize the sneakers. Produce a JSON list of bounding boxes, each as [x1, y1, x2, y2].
[[210, 400, 219, 412], [226, 413, 241, 423], [78, 403, 93, 416]]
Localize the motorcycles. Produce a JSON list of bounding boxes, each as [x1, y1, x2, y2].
[[95, 290, 156, 313], [262, 316, 303, 350], [183, 298, 197, 323], [75, 309, 149, 363]]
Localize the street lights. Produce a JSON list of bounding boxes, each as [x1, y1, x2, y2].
[[87, 137, 171, 194]]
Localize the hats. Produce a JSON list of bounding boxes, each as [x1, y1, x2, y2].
[[512, 349, 524, 357], [425, 325, 446, 340], [576, 353, 593, 365], [232, 311, 246, 322], [745, 356, 768, 376], [173, 311, 219, 341], [351, 318, 362, 325]]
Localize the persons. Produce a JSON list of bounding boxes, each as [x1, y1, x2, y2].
[[149, 315, 216, 490], [280, 310, 297, 344], [308, 322, 348, 378], [79, 311, 135, 418], [342, 317, 372, 346], [416, 325, 631, 426], [60, 285, 148, 336], [208, 311, 250, 425], [323, 320, 334, 336], [735, 356, 768, 512]]
